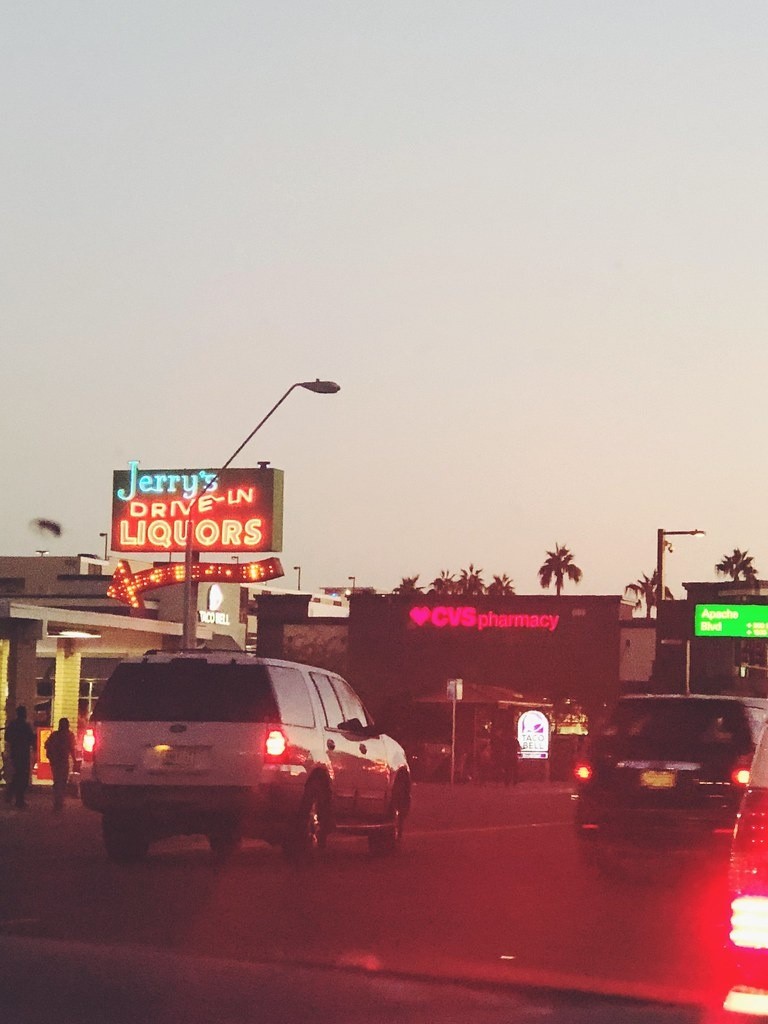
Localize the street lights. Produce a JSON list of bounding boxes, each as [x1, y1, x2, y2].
[[349, 576, 356, 594], [655, 529, 708, 612], [293, 566, 300, 590], [100, 533, 108, 561], [446, 677, 464, 785], [178, 375, 340, 651]]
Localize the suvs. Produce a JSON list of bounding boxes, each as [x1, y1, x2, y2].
[[571, 692, 767, 881], [78, 649, 411, 865]]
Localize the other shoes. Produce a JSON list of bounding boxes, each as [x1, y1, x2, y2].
[[3, 797, 15, 804], [15, 800, 30, 810]]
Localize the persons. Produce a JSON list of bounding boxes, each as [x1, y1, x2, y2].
[[43, 718, 77, 812], [2, 706, 37, 807], [503, 731, 522, 785]]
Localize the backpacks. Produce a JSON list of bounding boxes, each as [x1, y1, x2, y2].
[[46, 730, 71, 760]]
